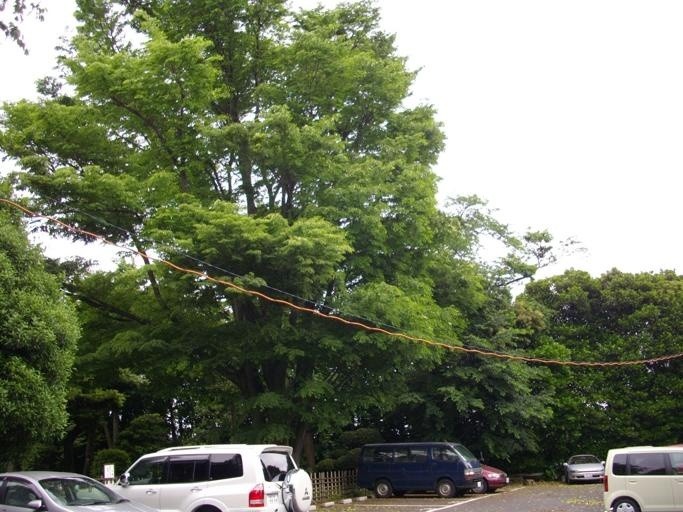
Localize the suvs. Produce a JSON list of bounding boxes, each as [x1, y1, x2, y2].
[[108, 444, 313, 512]]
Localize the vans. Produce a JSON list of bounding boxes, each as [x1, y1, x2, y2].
[[358, 441, 481, 498], [604, 445, 683, 512]]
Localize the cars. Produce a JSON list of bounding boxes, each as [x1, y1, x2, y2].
[[413, 450, 507, 494], [562, 454, 606, 483], [1, 470, 149, 511]]
